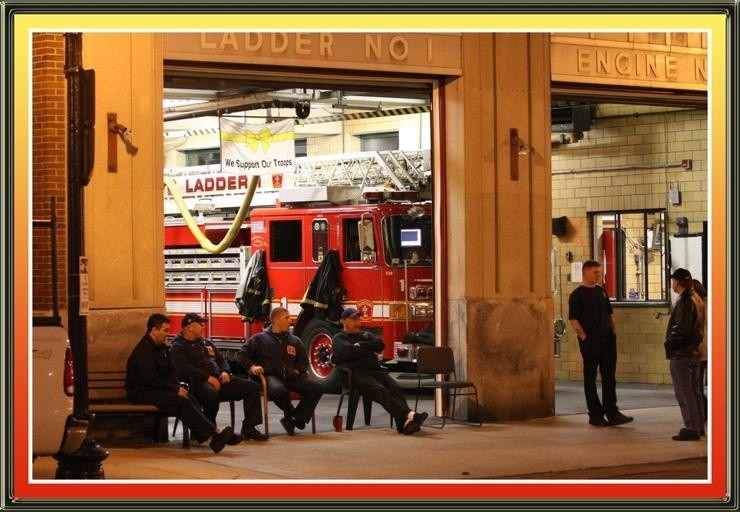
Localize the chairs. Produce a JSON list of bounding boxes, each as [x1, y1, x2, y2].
[[330, 361, 394, 430], [252, 368, 316, 435], [414, 345, 483, 429]]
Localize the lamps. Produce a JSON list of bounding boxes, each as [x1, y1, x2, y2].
[[510, 128, 529, 180], [106, 112, 134, 174]]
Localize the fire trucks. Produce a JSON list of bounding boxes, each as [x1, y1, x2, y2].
[[163, 150, 435, 390]]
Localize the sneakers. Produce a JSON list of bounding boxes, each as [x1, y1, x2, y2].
[[589, 412, 633, 426], [240, 427, 268, 441], [403, 413, 428, 435], [672, 429, 700, 441], [210, 426, 241, 454], [280, 406, 305, 436]]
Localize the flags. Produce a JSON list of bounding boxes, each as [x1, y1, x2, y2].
[[219, 116, 296, 174]]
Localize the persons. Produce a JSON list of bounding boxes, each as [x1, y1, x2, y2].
[[663, 267, 707, 442], [169, 312, 270, 448], [331, 307, 429, 434], [126, 311, 246, 453], [566, 259, 634, 427], [690, 278, 708, 422], [234, 306, 324, 435]]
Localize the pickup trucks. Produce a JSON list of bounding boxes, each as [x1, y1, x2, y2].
[[33, 314, 89, 458]]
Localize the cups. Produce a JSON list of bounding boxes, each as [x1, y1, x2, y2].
[[334, 416, 343, 432]]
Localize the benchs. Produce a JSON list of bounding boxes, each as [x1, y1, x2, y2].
[[88, 360, 238, 449]]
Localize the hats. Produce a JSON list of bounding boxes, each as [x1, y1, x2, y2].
[[668, 269, 692, 280], [342, 308, 362, 319], [182, 313, 209, 327]]
